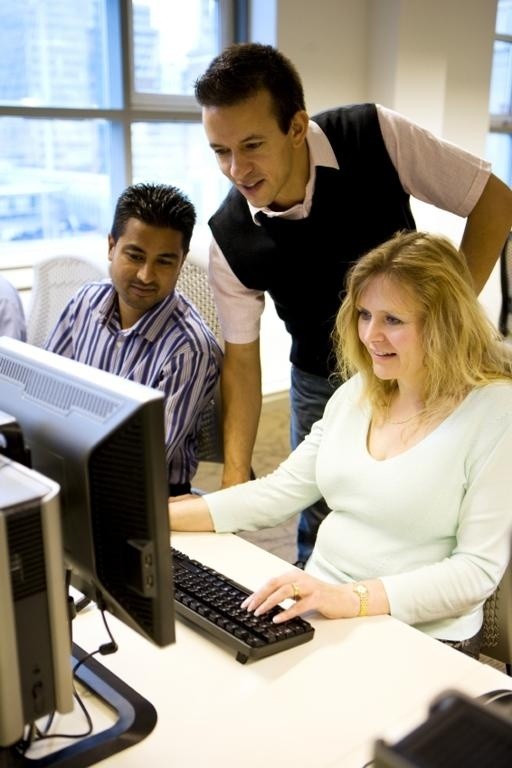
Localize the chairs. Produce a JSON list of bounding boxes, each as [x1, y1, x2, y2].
[[179, 257, 225, 463], [26, 256, 103, 348], [462, 557, 512, 680]]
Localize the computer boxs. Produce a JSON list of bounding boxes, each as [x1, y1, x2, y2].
[[0, 453, 73, 749]]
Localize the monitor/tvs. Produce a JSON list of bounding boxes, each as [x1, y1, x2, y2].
[[0, 337, 177, 768]]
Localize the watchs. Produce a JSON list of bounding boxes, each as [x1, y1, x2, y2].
[[346, 578, 371, 619]]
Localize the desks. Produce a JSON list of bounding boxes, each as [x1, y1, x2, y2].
[[1, 532, 512, 768]]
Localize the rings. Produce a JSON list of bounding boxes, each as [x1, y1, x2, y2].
[[291, 582, 301, 598]]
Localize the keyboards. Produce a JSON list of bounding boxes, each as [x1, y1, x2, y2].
[[170, 547, 316, 664]]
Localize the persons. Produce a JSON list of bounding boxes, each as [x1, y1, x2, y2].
[[0, 282, 29, 348], [192, 41, 512, 568], [37, 178, 222, 499], [171, 229, 512, 664]]
